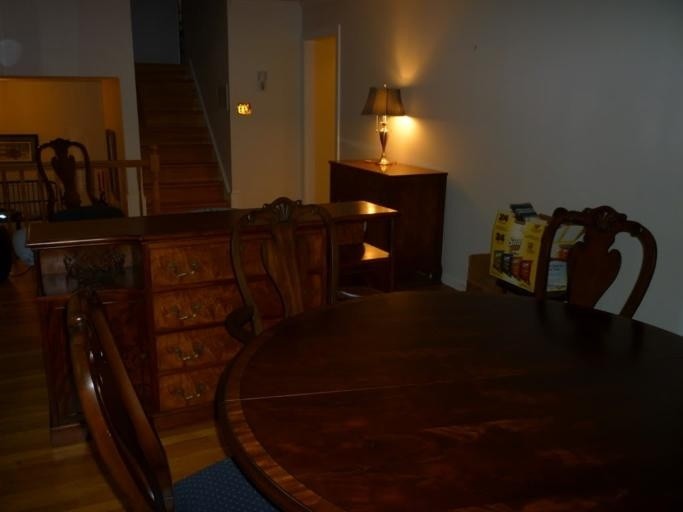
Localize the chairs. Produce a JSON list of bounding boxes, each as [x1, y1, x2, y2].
[[230, 196, 340, 335], [531, 202, 660, 321], [33, 136, 125, 221], [63, 285, 277, 510]]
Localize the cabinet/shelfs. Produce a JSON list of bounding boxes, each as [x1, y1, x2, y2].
[[329, 167, 447, 284]]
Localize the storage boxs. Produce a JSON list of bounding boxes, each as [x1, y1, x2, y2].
[[487, 206, 586, 295]]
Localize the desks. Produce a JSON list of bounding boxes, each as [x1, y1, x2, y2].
[[210, 288, 682, 509]]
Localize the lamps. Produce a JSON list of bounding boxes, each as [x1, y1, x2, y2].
[[360, 85, 409, 167]]
